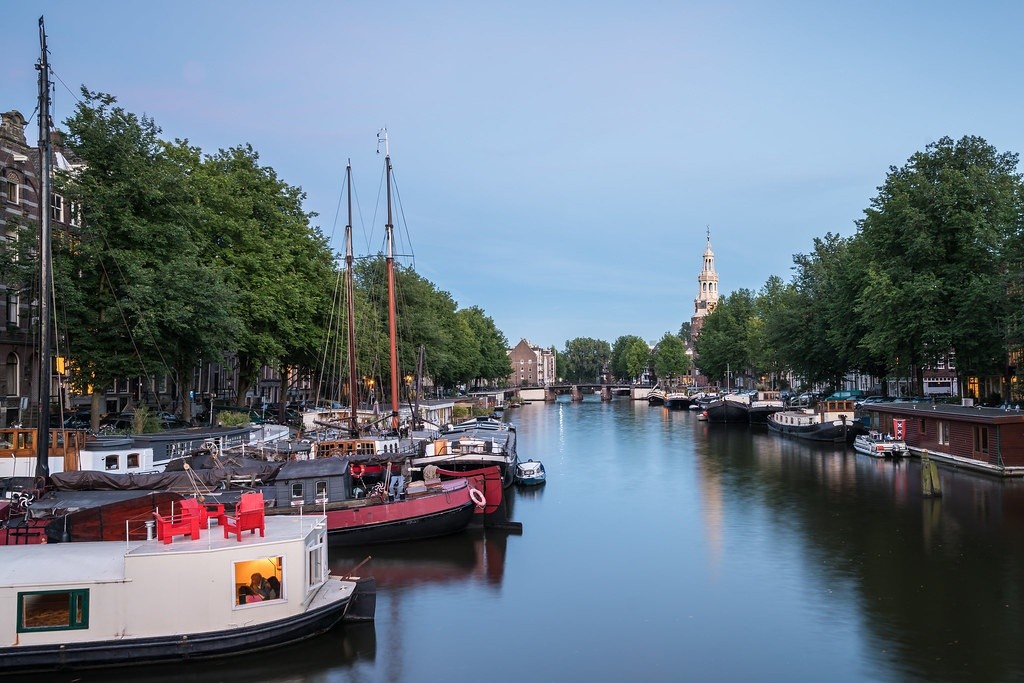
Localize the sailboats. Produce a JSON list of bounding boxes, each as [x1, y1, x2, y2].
[[0, 127, 519, 553], [1, 14, 377, 683]]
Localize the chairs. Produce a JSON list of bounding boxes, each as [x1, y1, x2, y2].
[[151, 511, 201, 546], [179, 497, 226, 530], [223, 491, 266, 541]]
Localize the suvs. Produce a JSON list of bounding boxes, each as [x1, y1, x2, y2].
[[100, 412, 151, 434], [50, 411, 91, 434]]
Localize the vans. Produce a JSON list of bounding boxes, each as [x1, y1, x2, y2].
[[799, 392, 821, 402], [824, 388, 864, 405]]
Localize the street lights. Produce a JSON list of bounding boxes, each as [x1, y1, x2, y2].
[[209, 393, 216, 423], [948, 369, 955, 401]]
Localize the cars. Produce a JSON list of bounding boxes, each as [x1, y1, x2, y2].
[[842, 394, 909, 410], [249, 402, 279, 409], [146, 412, 178, 429]]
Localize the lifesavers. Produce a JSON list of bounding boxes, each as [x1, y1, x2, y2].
[[350, 463, 365, 478], [469, 488, 487, 508]]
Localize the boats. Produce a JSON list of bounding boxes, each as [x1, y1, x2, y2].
[[766, 401, 861, 444], [646, 362, 783, 423], [515, 459, 546, 487], [511, 403, 521, 408], [852, 431, 911, 458]]
